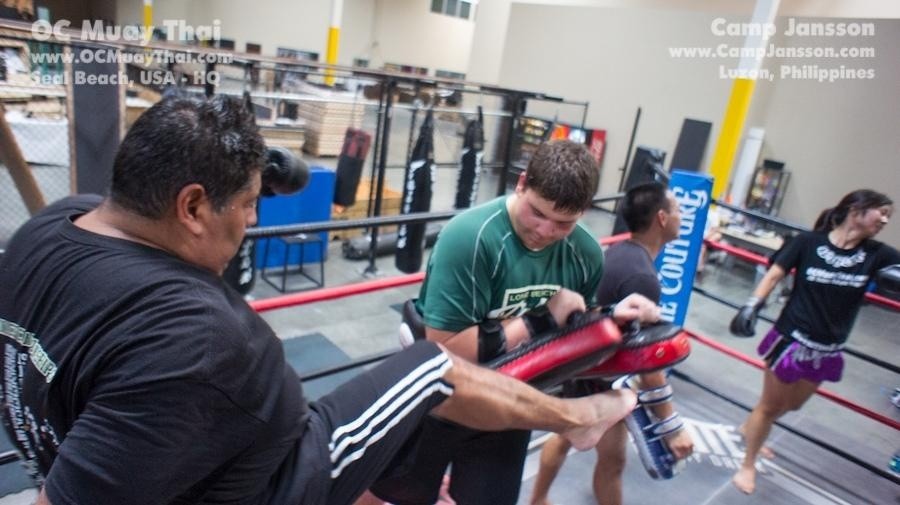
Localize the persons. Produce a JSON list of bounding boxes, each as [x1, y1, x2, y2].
[[729, 190, 900, 494]]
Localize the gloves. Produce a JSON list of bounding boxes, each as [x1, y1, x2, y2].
[[261, 146, 308, 197], [730, 296, 765, 337]]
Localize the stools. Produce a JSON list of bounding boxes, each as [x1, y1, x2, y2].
[[259, 231, 325, 295]]
